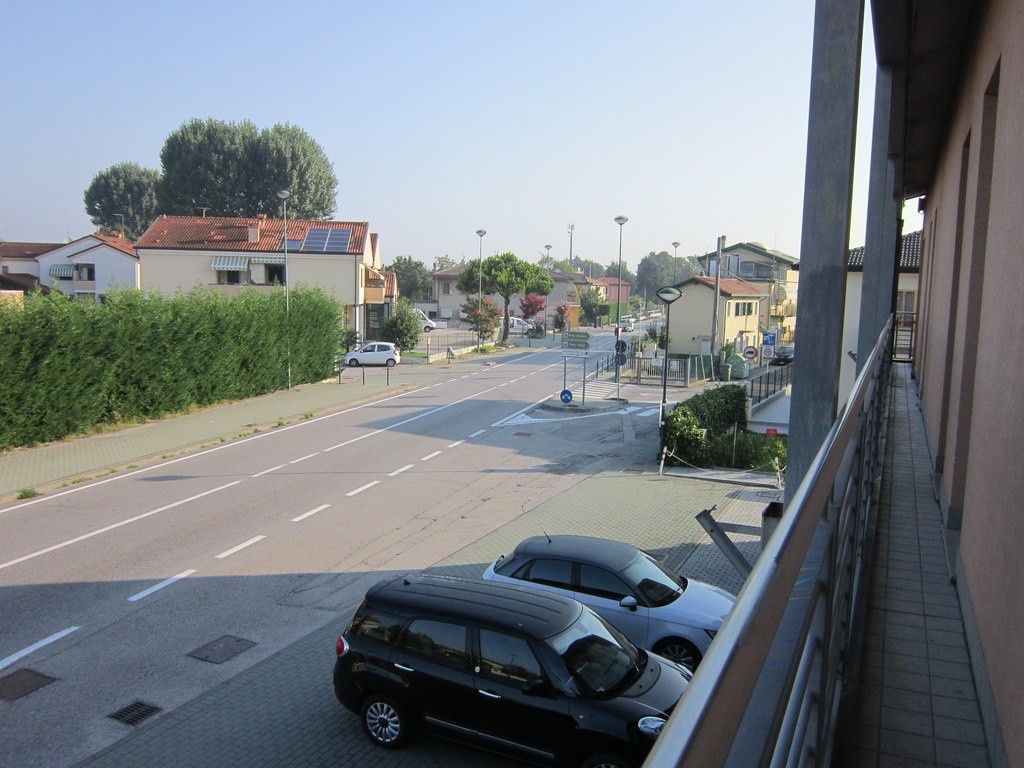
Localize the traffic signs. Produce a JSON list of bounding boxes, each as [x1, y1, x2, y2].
[[562, 332, 592, 342], [560, 341, 592, 351], [561, 349, 591, 358]]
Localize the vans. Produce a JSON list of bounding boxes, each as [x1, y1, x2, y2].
[[496, 316, 534, 335], [407, 309, 436, 334]]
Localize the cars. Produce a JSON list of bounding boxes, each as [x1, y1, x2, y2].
[[344, 342, 401, 367], [485, 532, 737, 674], [770, 346, 795, 364], [620, 320, 634, 332]]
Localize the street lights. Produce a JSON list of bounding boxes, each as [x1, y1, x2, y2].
[[672, 240, 681, 287], [277, 189, 292, 392], [476, 229, 487, 353], [544, 243, 553, 337], [656, 285, 682, 435], [614, 214, 629, 382]]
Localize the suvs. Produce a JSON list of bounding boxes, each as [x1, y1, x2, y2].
[[332, 571, 700, 768]]
